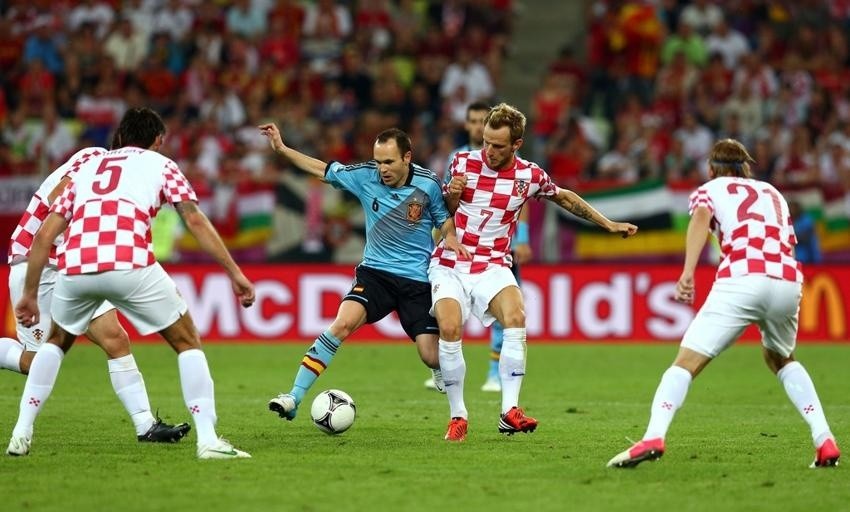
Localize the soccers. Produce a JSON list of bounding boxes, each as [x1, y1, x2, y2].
[[311, 389, 356, 435]]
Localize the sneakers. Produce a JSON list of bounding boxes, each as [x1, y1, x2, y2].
[[5, 434, 32, 457], [135, 418, 191, 443], [266, 392, 297, 421], [432, 367, 446, 394], [495, 404, 539, 434], [443, 417, 469, 442], [807, 438, 842, 469], [606, 436, 665, 469], [479, 378, 501, 392], [193, 438, 252, 460]]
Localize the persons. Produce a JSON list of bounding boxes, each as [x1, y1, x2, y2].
[[0, 130, 191, 444], [258, 123, 473, 422], [424, 102, 505, 392], [428, 103, 639, 440], [606, 140, 841, 469], [7, 106, 255, 459]]
